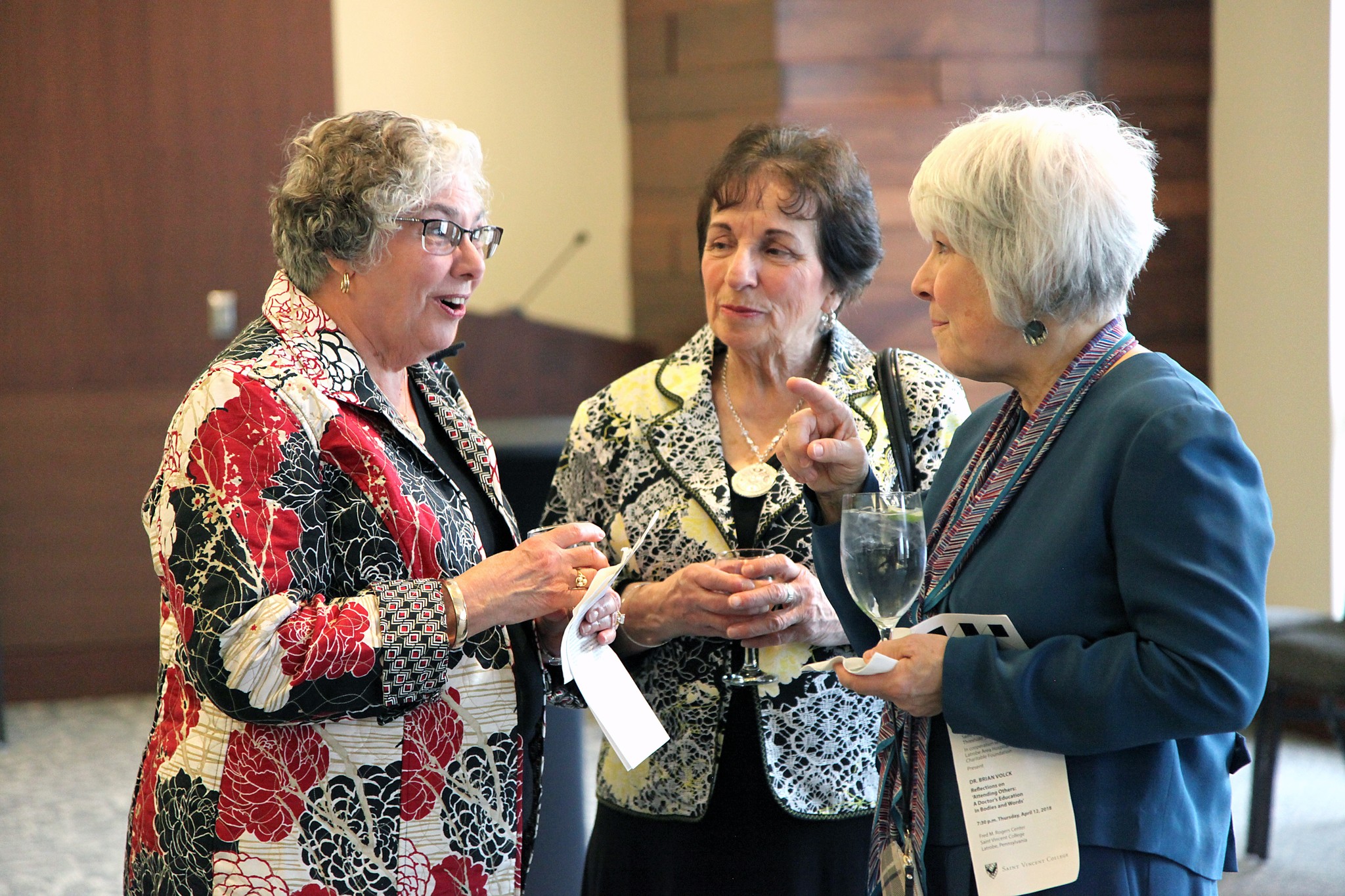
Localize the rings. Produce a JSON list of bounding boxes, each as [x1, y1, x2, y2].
[[783, 583, 794, 604], [576, 570, 586, 587], [615, 611, 625, 626]]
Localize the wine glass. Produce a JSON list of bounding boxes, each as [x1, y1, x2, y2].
[[526, 520, 597, 666], [839, 492, 927, 642], [711, 548, 779, 687]]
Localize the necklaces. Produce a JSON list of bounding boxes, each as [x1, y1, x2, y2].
[[720, 344, 826, 499], [370, 366, 426, 444]]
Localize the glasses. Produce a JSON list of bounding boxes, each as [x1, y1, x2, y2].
[[390, 217, 503, 259]]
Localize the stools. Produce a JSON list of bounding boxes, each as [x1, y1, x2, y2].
[[1245, 620, 1345, 859]]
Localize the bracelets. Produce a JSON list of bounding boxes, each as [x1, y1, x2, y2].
[[539, 642, 563, 666], [619, 581, 671, 647], [438, 578, 468, 650]]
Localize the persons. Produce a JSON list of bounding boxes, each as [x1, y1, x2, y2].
[[538, 125, 972, 896], [777, 98, 1273, 896], [121, 110, 621, 896]]
